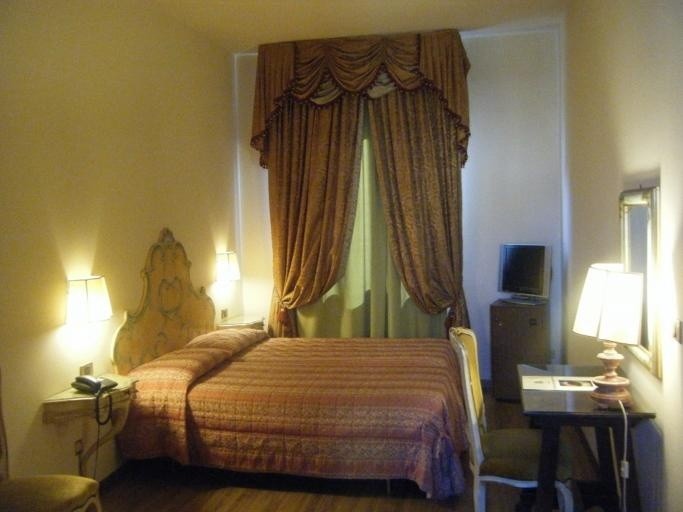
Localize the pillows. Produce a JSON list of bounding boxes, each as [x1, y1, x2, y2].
[[118, 347, 232, 467]]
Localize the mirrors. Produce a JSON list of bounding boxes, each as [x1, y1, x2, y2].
[[618, 185, 664, 379]]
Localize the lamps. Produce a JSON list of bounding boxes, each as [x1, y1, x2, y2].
[[62, 273, 113, 327], [572, 261, 644, 411], [209, 248, 243, 285]]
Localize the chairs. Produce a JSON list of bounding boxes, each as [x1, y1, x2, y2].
[[0, 414, 103, 512], [448, 319, 605, 512]]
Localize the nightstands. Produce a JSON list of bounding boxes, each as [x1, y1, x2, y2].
[[44, 374, 139, 477], [218, 316, 267, 333]]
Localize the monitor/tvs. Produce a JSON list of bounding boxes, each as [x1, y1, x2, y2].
[[495, 242, 553, 307]]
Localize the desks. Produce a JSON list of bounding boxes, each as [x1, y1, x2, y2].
[[520, 366, 656, 511]]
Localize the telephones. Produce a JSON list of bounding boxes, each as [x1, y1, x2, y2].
[[74, 378, 118, 425]]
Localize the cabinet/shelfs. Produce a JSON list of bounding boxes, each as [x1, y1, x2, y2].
[[490, 298, 549, 403]]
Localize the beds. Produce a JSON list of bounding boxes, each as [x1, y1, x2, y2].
[[109, 225, 465, 504]]
